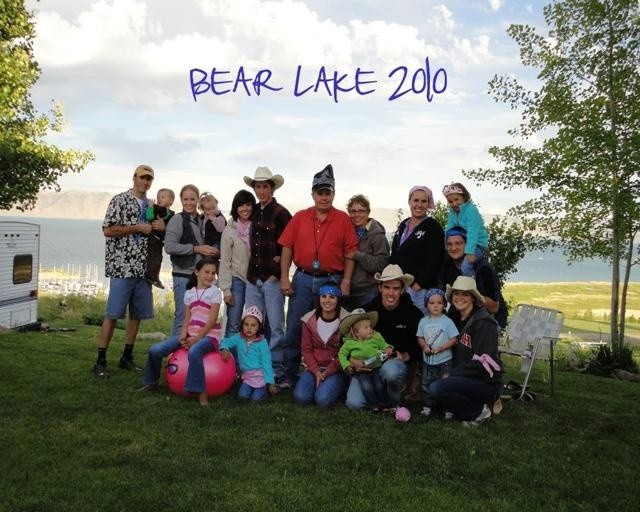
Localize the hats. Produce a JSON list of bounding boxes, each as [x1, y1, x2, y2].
[[134, 165, 154, 180], [446, 276, 485, 307], [312, 164, 335, 193], [243, 167, 284, 189], [339, 308, 379, 338], [374, 264, 415, 287]]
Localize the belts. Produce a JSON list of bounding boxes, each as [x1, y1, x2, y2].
[[297, 267, 341, 279]]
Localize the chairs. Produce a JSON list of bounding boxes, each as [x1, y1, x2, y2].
[[498, 303, 566, 400]]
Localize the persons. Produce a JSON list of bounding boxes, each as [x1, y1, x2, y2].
[[91, 164, 508, 421]]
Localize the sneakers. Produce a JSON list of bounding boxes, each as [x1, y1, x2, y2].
[[441, 411, 454, 424], [144, 271, 164, 288], [118, 356, 144, 374], [463, 408, 491, 429], [92, 360, 110, 379], [420, 406, 433, 423]]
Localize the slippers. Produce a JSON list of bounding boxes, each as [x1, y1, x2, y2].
[[504, 380, 536, 403]]
